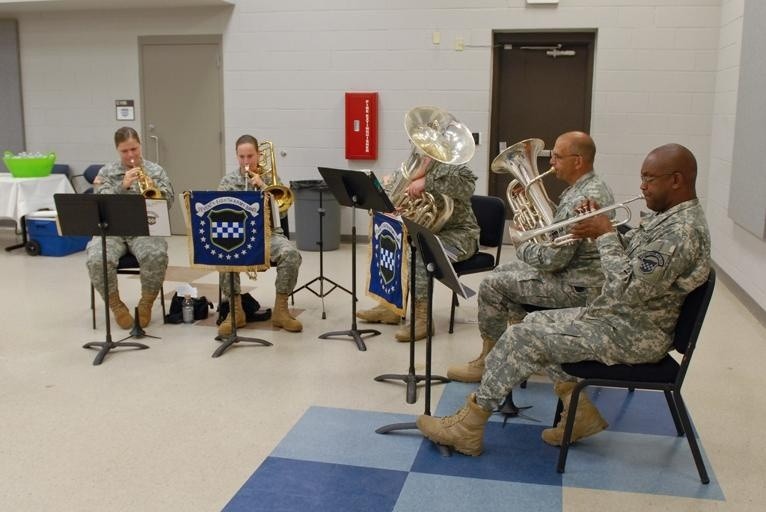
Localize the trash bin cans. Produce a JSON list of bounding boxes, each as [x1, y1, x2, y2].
[[290, 180, 341, 251]]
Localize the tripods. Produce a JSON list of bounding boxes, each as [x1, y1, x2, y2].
[[373, 246, 452, 404], [289, 191, 359, 319], [82, 229, 149, 366], [211, 272, 273, 357], [318, 200, 381, 350], [375, 271, 451, 458]]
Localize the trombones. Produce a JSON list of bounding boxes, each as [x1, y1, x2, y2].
[[244, 140, 293, 212]]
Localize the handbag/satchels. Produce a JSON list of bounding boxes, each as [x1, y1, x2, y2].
[[215, 293, 272, 325], [165, 292, 214, 325]]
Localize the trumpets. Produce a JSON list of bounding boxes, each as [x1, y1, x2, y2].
[[509, 193, 646, 251], [129, 158, 161, 198]]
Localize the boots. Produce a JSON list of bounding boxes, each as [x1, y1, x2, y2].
[[446, 339, 497, 383], [108, 291, 133, 328], [218, 295, 245, 337], [416, 392, 493, 456], [542, 380, 607, 447], [272, 292, 302, 332], [395, 298, 434, 341], [137, 290, 158, 327], [357, 304, 401, 323]]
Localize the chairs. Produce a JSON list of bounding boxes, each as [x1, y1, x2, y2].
[[68, 164, 102, 196], [49, 164, 71, 178], [553, 266, 714, 484], [440, 193, 505, 335], [216, 210, 291, 320], [90, 241, 165, 331]]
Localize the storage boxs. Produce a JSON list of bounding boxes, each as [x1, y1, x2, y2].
[[25, 212, 91, 256]]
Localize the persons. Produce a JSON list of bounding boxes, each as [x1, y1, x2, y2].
[[448, 130, 616, 383], [359, 150, 480, 343], [214, 133, 301, 334], [83, 127, 175, 331], [415, 142, 712, 455]]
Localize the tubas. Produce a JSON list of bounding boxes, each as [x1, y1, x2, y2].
[[491, 138, 559, 247], [368, 105, 476, 233]]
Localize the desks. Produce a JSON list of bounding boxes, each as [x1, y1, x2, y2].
[[0, 173, 69, 251]]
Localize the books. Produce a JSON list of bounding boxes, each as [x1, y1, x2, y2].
[[355, 168, 395, 214], [178, 193, 187, 225], [435, 235, 468, 299], [269, 194, 281, 228], [54, 211, 62, 236], [145, 198, 172, 237]]
